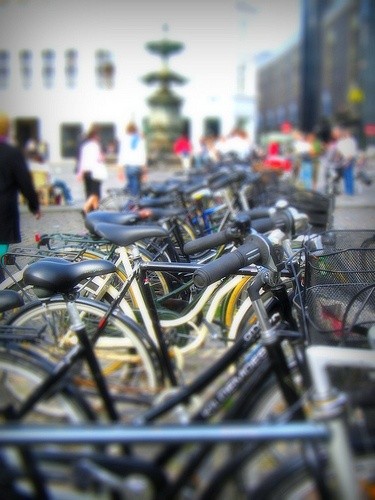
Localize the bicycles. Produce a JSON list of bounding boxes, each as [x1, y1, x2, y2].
[[0, 158, 373, 499]]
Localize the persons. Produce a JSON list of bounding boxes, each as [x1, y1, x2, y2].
[[75, 130, 105, 216], [0, 113, 41, 268], [170, 119, 374, 204], [8, 135, 117, 164], [118, 122, 147, 196], [30, 155, 72, 204]]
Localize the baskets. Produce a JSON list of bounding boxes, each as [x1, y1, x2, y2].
[[290, 281, 375, 345], [291, 190, 329, 233], [302, 222, 375, 289]]
